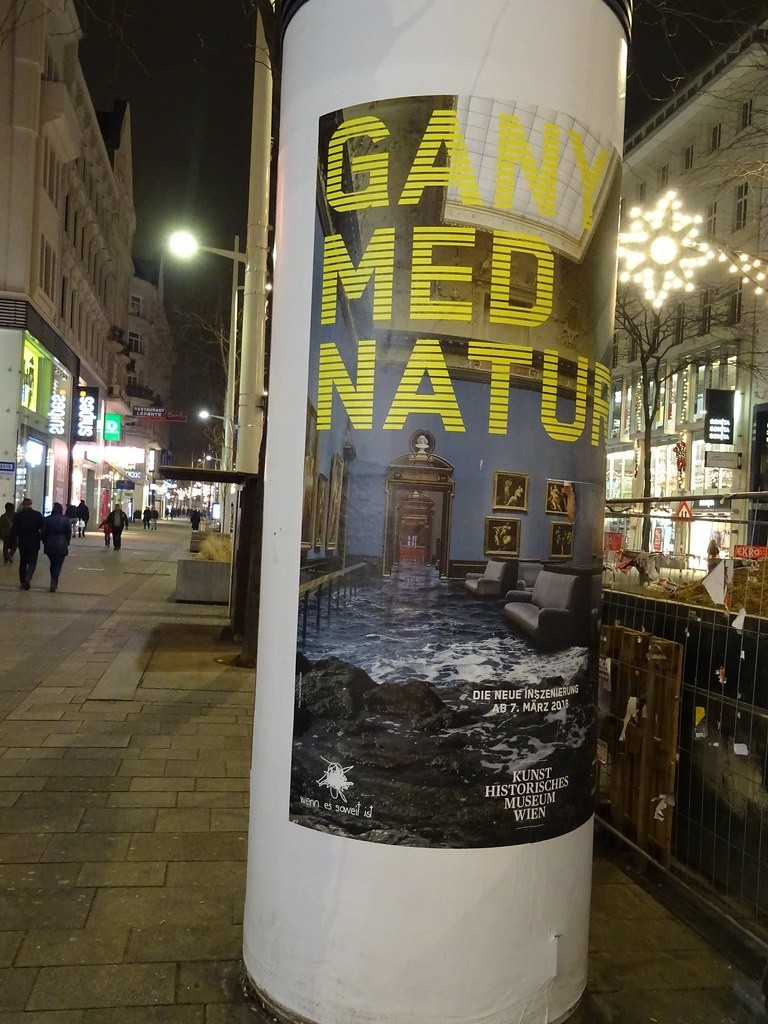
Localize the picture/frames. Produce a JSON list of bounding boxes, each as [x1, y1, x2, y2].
[[550, 520, 574, 559], [485, 516, 521, 557], [302, 398, 321, 551], [492, 471, 528, 512], [325, 452, 344, 551], [545, 481, 570, 515], [315, 473, 329, 548]]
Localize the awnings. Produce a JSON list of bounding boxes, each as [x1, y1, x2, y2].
[[105, 460, 142, 486]]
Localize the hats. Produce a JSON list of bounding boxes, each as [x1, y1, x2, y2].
[[5, 503, 14, 511], [23, 498, 32, 506], [53, 502, 63, 512]]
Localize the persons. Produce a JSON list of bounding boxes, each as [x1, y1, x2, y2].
[[16, 498, 72, 592], [166, 506, 206, 531], [0, 503, 18, 562], [143, 507, 159, 530], [65, 500, 90, 537], [99, 504, 128, 550], [707, 540, 720, 558]]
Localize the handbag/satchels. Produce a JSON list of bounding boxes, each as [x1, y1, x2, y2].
[[78, 518, 85, 527]]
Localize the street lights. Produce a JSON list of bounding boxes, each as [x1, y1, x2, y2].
[[219, 235, 272, 506], [165, 230, 269, 468]]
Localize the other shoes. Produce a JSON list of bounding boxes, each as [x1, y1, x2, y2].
[[25, 579, 31, 590], [78, 533, 81, 537], [6, 556, 14, 563], [4, 558, 8, 563], [82, 532, 85, 537], [50, 584, 56, 593]]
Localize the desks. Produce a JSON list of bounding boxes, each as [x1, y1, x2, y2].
[[297, 560, 367, 641]]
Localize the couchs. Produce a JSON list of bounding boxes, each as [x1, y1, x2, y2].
[[465, 561, 595, 648]]
